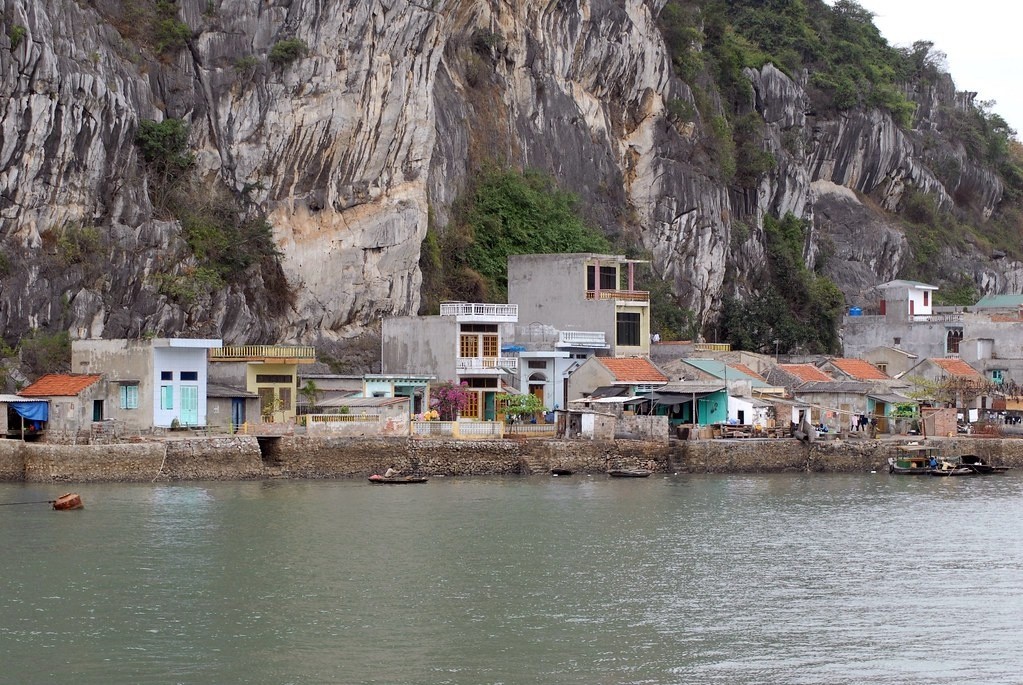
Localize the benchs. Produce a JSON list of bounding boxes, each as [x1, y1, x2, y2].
[[720, 424, 755, 438]]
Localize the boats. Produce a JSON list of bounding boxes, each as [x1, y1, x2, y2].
[[887, 444, 1015, 475], [368, 474, 428, 484], [605, 468, 652, 478]]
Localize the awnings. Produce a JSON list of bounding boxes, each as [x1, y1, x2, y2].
[[654, 385, 726, 425], [868, 394, 917, 404]]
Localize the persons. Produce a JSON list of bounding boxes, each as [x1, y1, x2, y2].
[[819, 423, 828, 432], [942, 460, 956, 471], [930, 457, 938, 467], [384, 466, 396, 478], [30, 424, 34, 431]]
